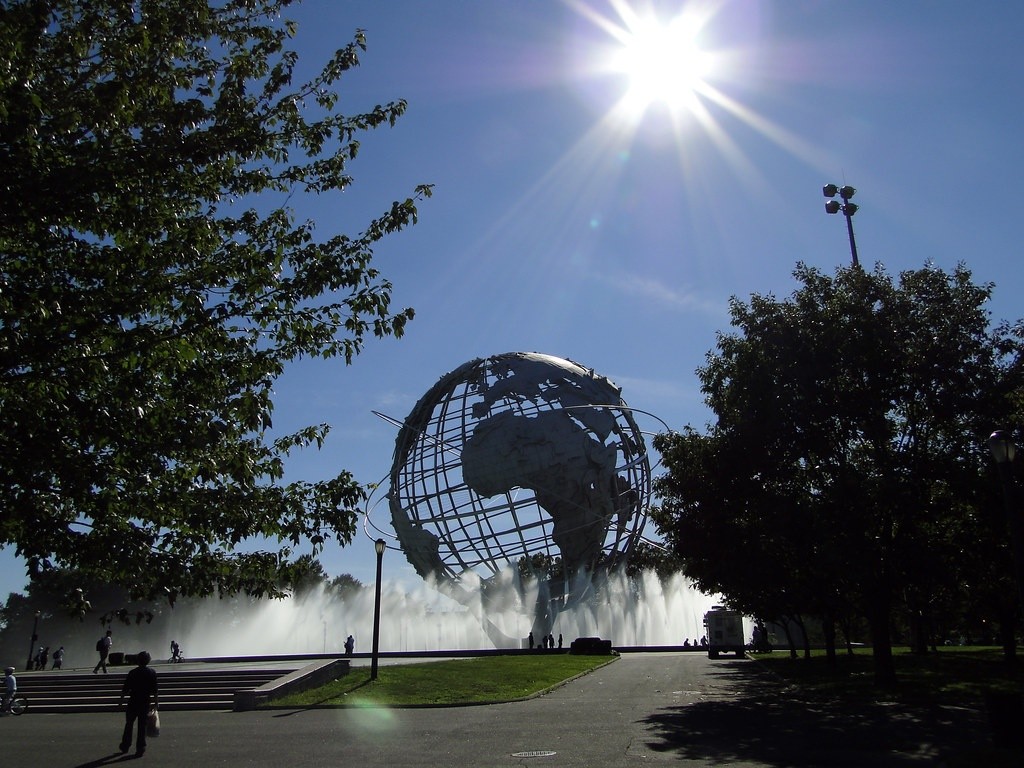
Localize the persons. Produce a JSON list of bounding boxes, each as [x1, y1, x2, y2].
[[34, 645, 65, 671], [529, 631, 563, 650], [684, 636, 708, 649], [752, 623, 773, 654], [0, 667, 16, 714], [171, 640, 182, 663], [344, 635, 354, 657], [93, 630, 113, 674], [118, 650, 159, 758]]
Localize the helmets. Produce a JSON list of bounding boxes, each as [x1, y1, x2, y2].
[[3, 667, 17, 672]]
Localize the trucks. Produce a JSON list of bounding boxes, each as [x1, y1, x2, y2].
[[703, 605, 746, 660]]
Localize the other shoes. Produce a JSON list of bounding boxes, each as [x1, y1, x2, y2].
[[119, 742, 129, 753], [93, 669, 98, 674], [135, 747, 145, 758]]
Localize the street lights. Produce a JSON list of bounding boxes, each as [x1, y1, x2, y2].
[[822, 181, 858, 268], [26, 611, 42, 671], [368, 538, 386, 681]]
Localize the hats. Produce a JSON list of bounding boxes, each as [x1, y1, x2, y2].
[[38, 646, 44, 650]]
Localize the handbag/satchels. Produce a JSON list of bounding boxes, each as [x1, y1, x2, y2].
[[146, 708, 160, 737]]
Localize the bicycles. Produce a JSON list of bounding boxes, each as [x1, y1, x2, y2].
[[0, 692, 29, 715], [168, 650, 185, 664]]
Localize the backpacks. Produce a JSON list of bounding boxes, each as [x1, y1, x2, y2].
[[96, 636, 108, 652]]
